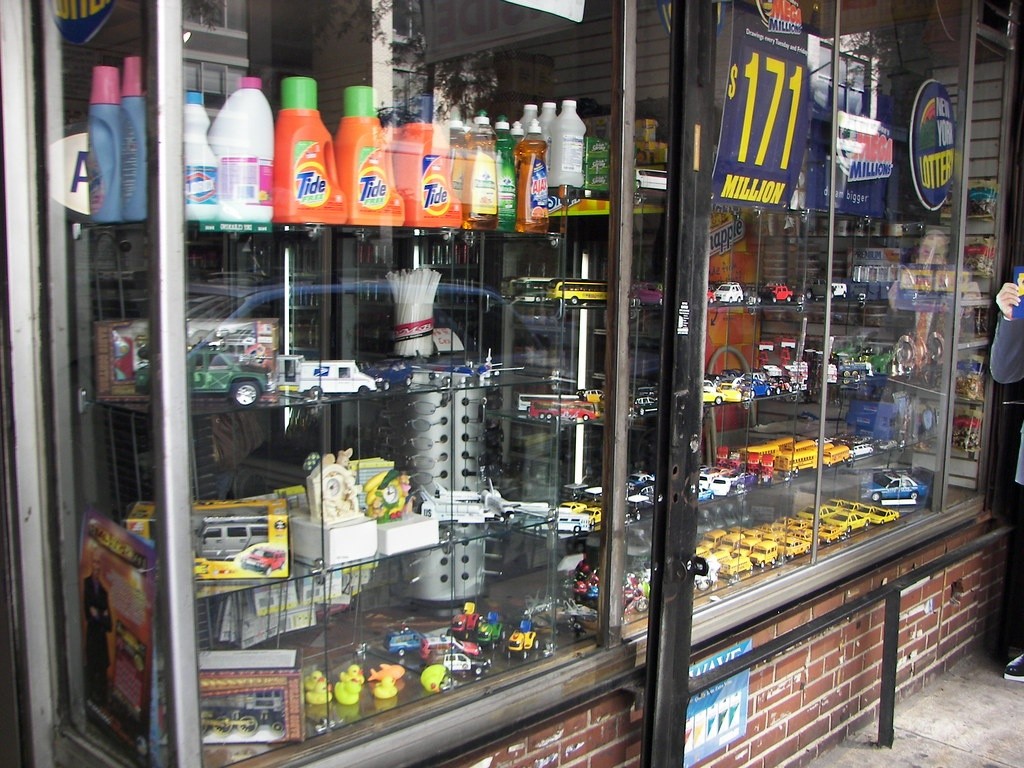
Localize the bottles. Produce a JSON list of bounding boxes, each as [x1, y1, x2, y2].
[[448, 99, 587, 235]]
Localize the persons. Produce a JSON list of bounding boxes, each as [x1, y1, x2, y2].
[[885, 232, 973, 358], [989, 266, 1024, 683]]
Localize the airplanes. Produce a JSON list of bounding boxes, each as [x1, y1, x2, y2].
[[474, 347, 526, 383], [414, 349, 474, 386]]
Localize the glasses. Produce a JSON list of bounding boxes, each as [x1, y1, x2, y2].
[[378, 396, 447, 557], [467, 390, 506, 580]]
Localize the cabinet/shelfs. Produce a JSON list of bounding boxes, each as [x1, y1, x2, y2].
[[484, 192, 986, 544], [77, 181, 613, 617]]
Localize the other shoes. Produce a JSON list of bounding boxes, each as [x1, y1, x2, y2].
[[1003, 653, 1024, 682]]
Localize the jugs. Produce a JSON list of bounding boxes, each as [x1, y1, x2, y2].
[[85, 56, 463, 230]]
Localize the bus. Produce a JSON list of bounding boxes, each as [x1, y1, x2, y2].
[[511, 277, 552, 304], [548, 278, 608, 306]]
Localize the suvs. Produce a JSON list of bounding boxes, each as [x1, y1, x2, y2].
[[242, 547, 287, 574], [707, 281, 797, 305], [296, 360, 378, 397], [130, 350, 274, 406]]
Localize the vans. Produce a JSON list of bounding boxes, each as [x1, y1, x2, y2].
[[195, 516, 269, 562]]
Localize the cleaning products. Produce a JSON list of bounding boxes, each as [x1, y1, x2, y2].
[[85, 55, 588, 235]]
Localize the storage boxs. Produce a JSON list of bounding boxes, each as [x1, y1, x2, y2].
[[290, 514, 379, 568], [376, 514, 439, 556], [126, 501, 296, 583], [156, 649, 302, 743], [94, 314, 279, 402]]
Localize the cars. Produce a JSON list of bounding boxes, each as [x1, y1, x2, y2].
[[380, 351, 897, 678], [629, 282, 663, 307], [858, 469, 931, 503], [364, 358, 415, 391]]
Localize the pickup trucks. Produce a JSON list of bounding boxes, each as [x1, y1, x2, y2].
[[804, 278, 847, 303]]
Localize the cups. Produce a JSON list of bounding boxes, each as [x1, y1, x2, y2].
[[393, 302, 436, 360]]
